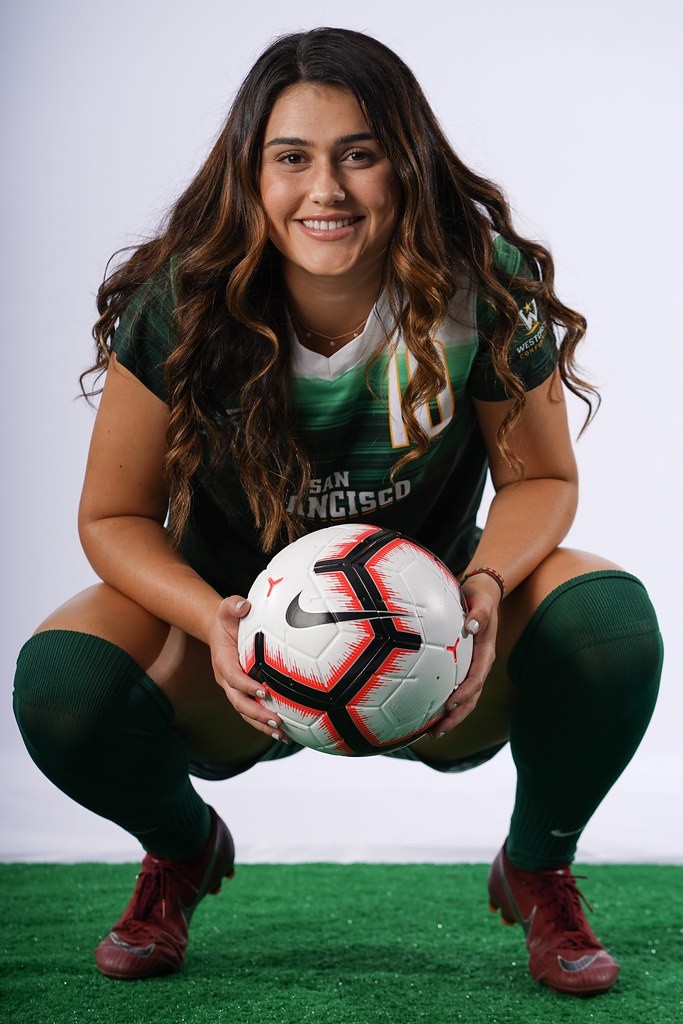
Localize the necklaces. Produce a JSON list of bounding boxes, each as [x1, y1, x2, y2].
[[288, 307, 367, 347]]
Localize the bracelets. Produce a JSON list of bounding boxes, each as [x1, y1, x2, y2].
[[460, 567, 507, 607]]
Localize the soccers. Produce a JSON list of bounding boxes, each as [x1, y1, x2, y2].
[[237, 525, 477, 760]]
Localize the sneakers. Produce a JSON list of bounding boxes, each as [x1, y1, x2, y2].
[[486, 840, 617, 997], [93, 809, 236, 981]]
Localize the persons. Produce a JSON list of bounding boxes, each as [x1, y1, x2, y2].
[[10, 26, 664, 999]]
